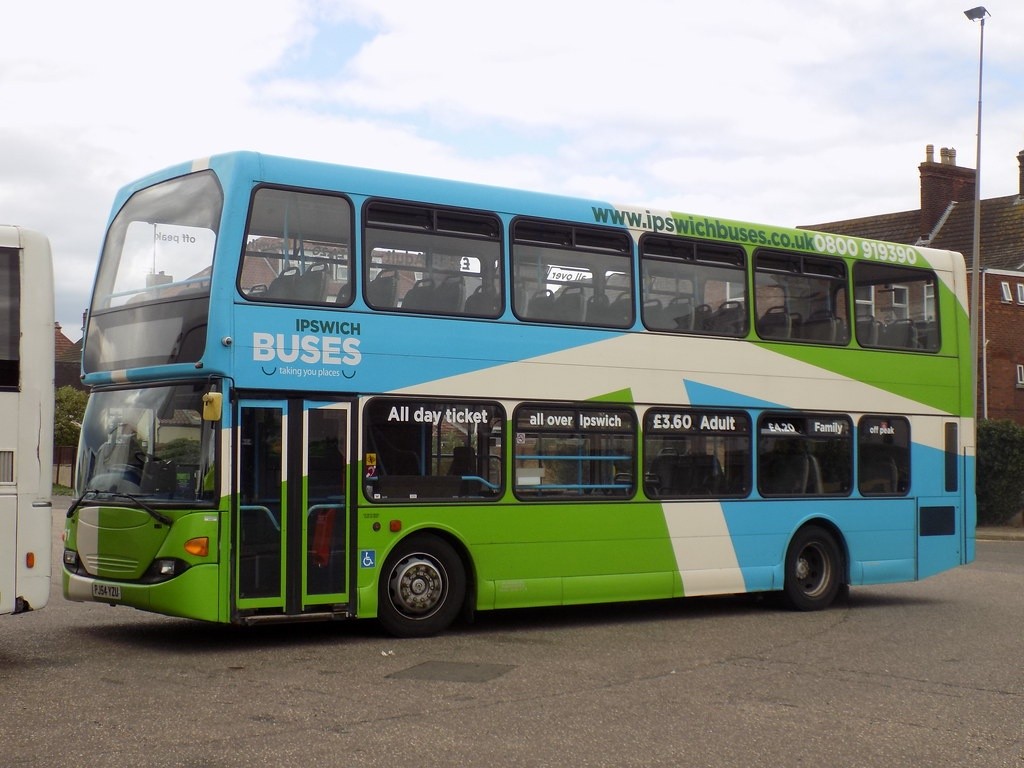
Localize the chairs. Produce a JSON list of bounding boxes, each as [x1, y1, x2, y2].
[[248, 262, 329, 305], [335, 266, 940, 352], [447, 447, 477, 494], [614, 446, 899, 496]]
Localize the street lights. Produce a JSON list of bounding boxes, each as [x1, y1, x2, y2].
[[964, 5, 992, 458]]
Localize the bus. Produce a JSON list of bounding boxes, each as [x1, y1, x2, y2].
[[58, 149, 981, 641], [1, 223, 59, 625]]
